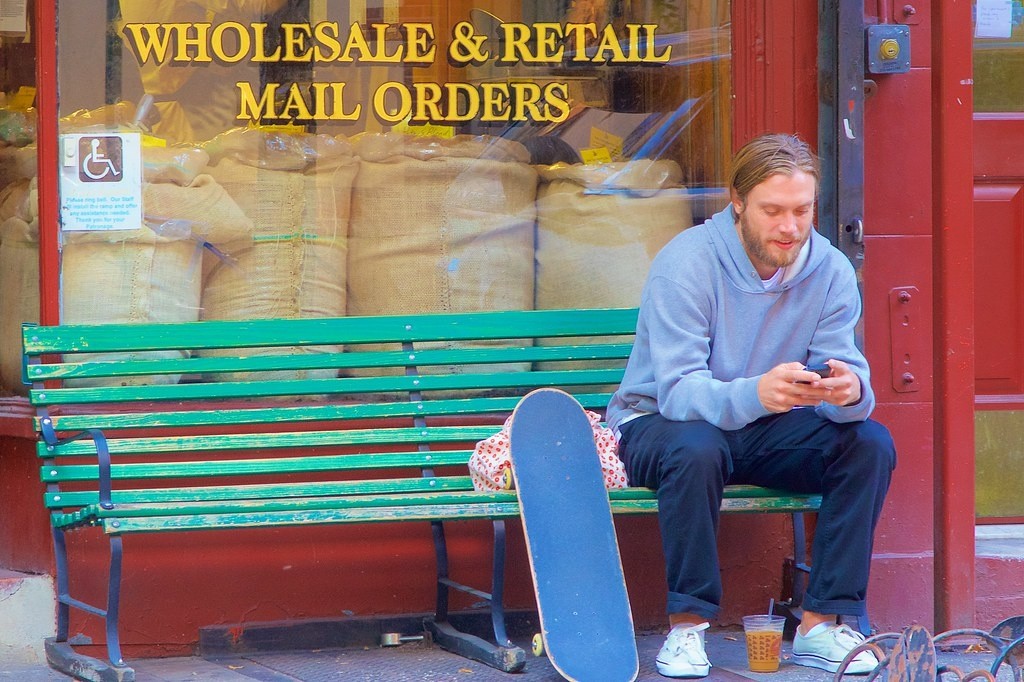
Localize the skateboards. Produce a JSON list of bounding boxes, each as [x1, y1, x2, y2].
[[503, 386, 641, 682]]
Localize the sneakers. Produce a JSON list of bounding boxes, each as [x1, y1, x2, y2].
[[792, 621, 878, 673], [656, 622, 712, 677]]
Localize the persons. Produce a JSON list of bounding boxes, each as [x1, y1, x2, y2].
[[605, 133, 897, 677]]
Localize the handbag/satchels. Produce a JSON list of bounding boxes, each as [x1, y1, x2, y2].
[[468, 411, 628, 492]]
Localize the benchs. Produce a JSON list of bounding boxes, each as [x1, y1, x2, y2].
[[21, 308, 822, 682]]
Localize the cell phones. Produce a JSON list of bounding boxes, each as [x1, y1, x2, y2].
[[797, 363, 832, 384]]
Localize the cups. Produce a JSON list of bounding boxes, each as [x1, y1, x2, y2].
[[742, 615, 786, 672]]
